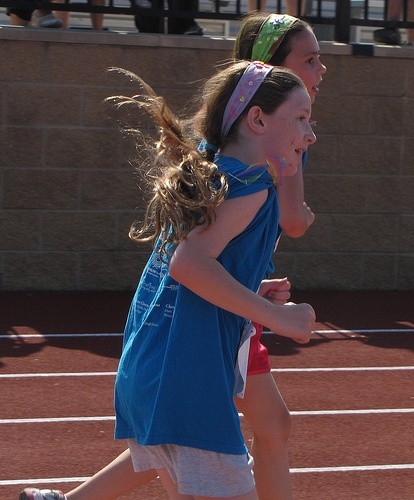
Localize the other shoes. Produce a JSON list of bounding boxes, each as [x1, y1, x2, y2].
[[31, 12, 64, 29], [18, 488, 65, 500], [183, 21, 203, 35], [373, 28, 402, 45]]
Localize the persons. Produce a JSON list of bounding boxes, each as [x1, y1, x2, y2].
[[5, 0, 106, 30], [388, 0, 414, 44], [129, 0, 203, 36], [17, 12, 327, 500], [114, 60, 317, 500], [248, 0, 307, 17]]
